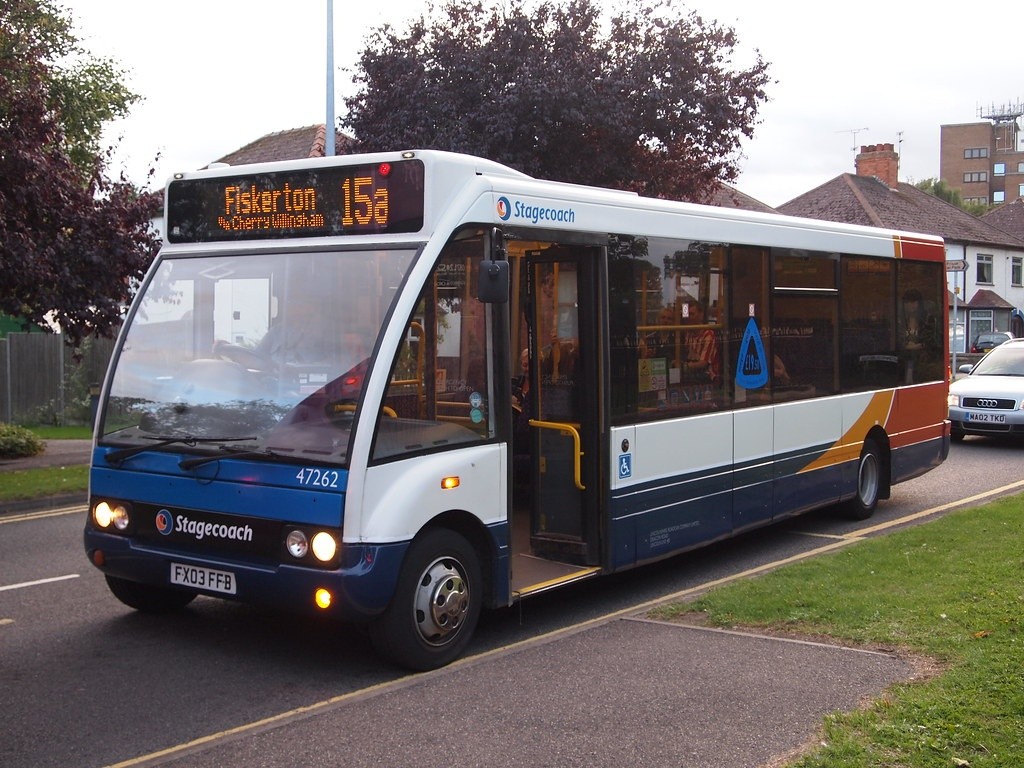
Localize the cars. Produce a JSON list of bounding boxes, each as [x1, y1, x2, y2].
[[970, 331, 1015, 354], [947, 337, 1024, 441]]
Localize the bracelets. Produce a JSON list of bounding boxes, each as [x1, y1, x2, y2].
[[685, 361, 691, 369]]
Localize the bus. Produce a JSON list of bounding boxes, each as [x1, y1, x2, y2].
[[83, 148, 951, 674]]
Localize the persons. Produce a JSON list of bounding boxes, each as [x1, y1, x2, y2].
[[453, 290, 941, 458], [211, 278, 338, 401]]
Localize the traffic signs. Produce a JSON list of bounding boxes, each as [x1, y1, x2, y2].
[[946, 259, 968, 273]]
